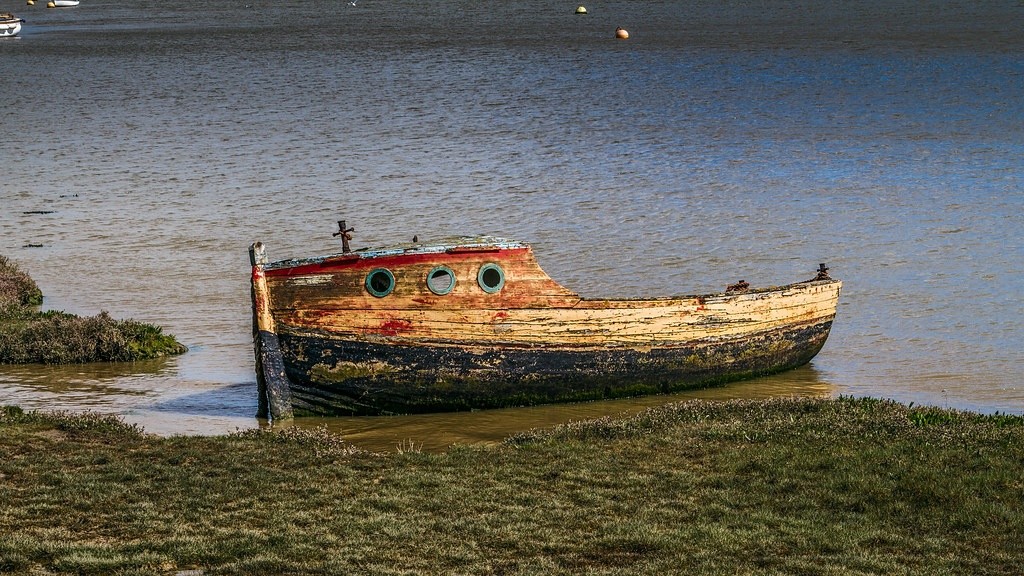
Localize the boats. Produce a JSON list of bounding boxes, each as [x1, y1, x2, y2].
[[247, 219, 844, 420], [0, 12, 27, 39], [53, 0, 80, 7]]
